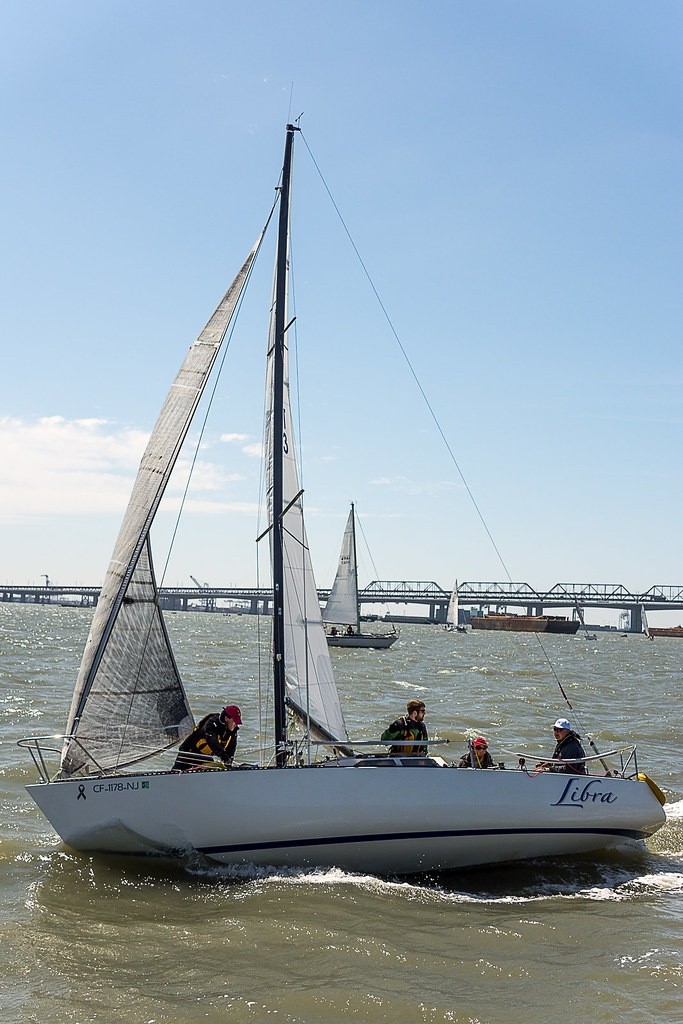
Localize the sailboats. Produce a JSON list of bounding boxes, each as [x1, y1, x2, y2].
[[321, 497, 399, 649], [444, 580, 468, 632], [641, 602, 654, 641], [22, 111, 664, 901], [575, 596, 597, 640]]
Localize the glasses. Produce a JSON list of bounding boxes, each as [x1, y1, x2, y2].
[[419, 709, 425, 713], [554, 728, 565, 732], [475, 745, 487, 750]]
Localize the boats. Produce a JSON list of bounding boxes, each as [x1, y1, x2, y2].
[[542, 615, 580, 634], [644, 627, 683, 637], [470, 616, 549, 632], [61, 597, 89, 608]]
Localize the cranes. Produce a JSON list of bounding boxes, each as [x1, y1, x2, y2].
[[189, 575, 216, 612]]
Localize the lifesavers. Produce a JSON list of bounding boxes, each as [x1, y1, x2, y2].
[[638, 772, 666, 806]]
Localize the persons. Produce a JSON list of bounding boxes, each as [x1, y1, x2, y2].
[[379, 699, 430, 758], [330, 626, 340, 635], [171, 704, 243, 772], [346, 624, 355, 635], [458, 736, 498, 768], [535, 717, 587, 776]]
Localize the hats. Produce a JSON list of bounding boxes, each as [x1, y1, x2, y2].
[[222, 704, 243, 724], [472, 737, 488, 748], [550, 718, 570, 730]]
